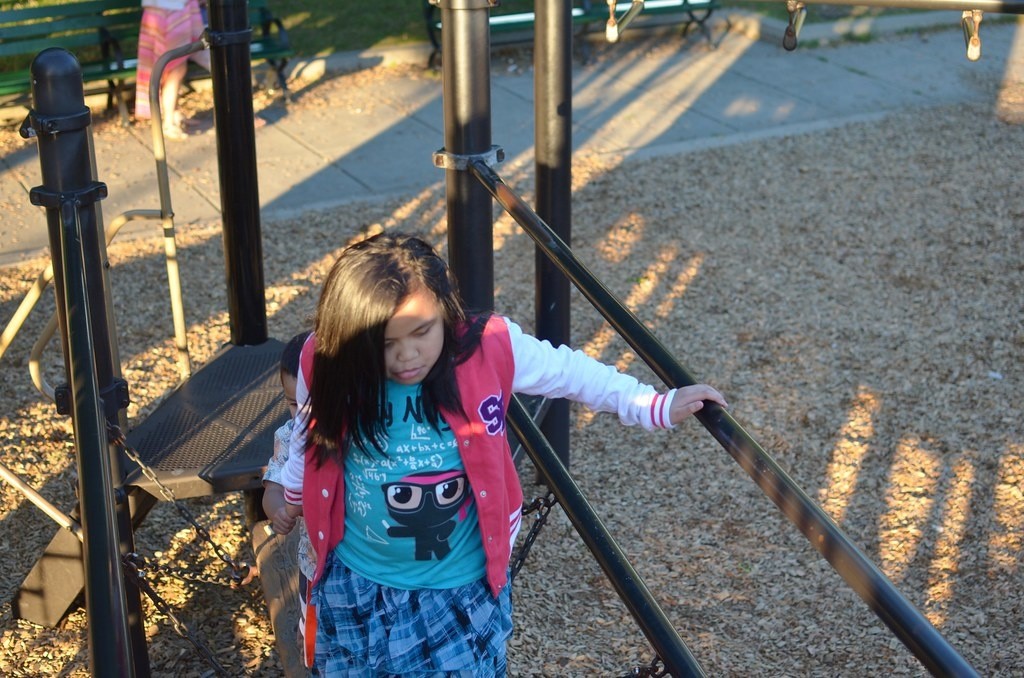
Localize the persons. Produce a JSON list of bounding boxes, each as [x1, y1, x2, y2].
[[133, 0, 212, 144], [259, 228, 731, 678]]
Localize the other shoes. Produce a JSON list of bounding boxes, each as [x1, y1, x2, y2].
[[254, 117, 266, 129], [161, 123, 188, 142]]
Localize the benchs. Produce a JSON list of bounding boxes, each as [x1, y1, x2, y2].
[[422, 0, 722, 70], [0, 0, 295, 126]]
